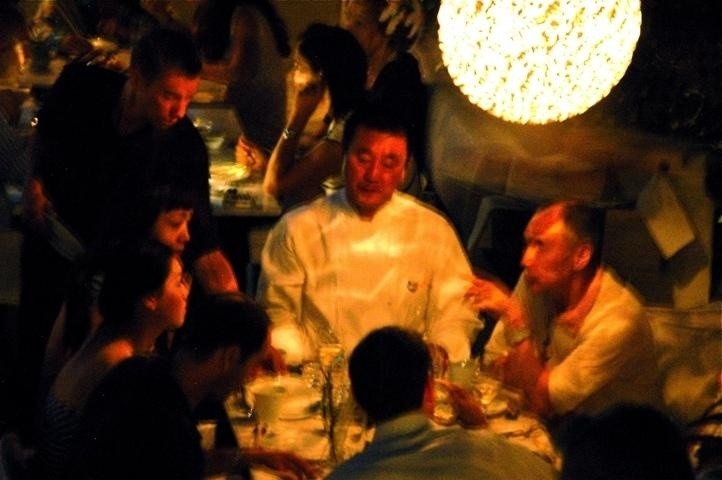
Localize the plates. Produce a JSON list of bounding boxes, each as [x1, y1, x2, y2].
[[227, 389, 320, 421], [432, 402, 508, 424], [208, 164, 253, 182]]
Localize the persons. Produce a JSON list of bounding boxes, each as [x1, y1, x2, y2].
[[1, 1, 722, 479]]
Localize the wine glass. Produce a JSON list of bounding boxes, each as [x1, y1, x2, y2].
[[474, 365, 501, 418]]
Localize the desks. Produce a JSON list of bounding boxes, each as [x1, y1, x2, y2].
[[1, 34, 282, 219]]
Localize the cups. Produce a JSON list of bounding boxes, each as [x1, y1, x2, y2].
[[252, 386, 288, 424], [448, 359, 477, 391]]
